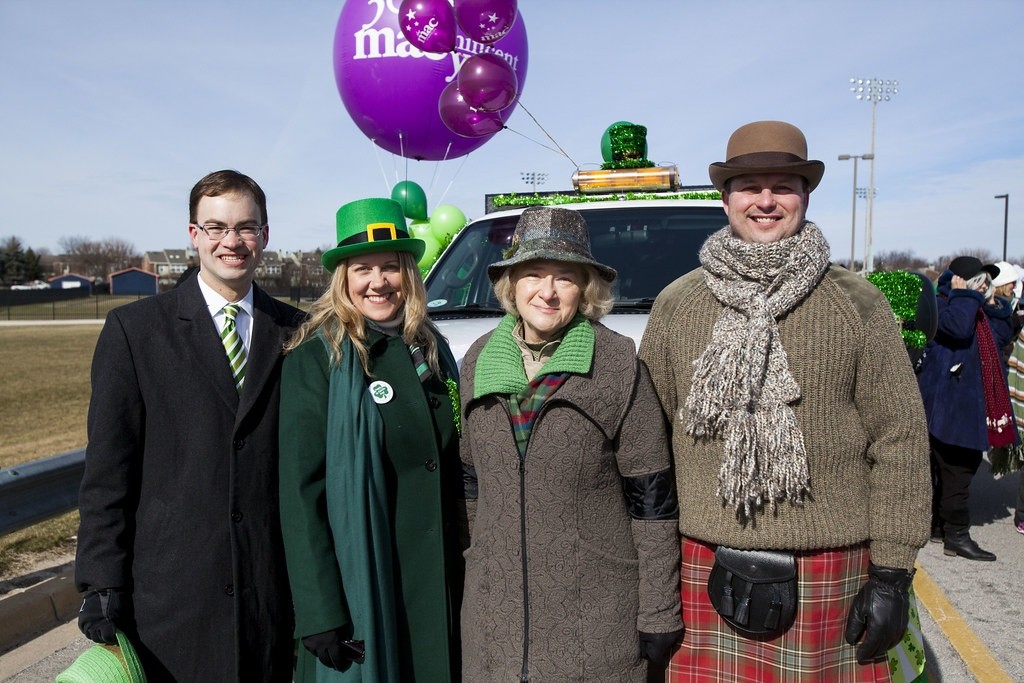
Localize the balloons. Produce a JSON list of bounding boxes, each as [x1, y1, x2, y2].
[[453, 0, 517, 38], [601, 121, 649, 163], [391, 180, 464, 270], [458, 53, 518, 112], [438, 80, 507, 138], [398, 0, 456, 54], [333, 0, 529, 160]]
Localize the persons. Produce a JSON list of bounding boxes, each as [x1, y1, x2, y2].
[[277, 203, 472, 683], [457, 207, 685, 683], [73, 168, 318, 683], [636, 119, 933, 683], [918, 256, 1024, 561]]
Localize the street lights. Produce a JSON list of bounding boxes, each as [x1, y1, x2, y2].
[[838, 154, 874, 272], [995, 193, 1008, 262], [850, 77, 899, 272]]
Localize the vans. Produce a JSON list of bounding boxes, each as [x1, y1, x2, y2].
[[423, 190, 729, 379]]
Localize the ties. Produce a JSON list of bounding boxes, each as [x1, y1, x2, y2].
[[219, 304, 249, 400]]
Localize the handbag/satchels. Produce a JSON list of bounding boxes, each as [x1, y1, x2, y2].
[[707, 548, 799, 641]]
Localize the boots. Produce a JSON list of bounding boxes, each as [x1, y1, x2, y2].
[[930, 502, 945, 539], [944, 509, 996, 561]]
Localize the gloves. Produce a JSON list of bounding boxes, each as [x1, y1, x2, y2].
[[301, 622, 356, 673], [78, 587, 125, 645], [845, 560, 917, 661], [637, 628, 686, 683]]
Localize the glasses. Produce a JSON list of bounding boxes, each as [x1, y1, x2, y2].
[[340, 638, 366, 664], [193, 221, 267, 241], [1011, 281, 1017, 287]]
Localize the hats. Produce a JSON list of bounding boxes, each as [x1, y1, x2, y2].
[[949, 256, 1000, 281], [709, 120, 825, 193], [991, 261, 1017, 287], [322, 198, 427, 273], [54, 631, 149, 683], [487, 206, 617, 288]]
